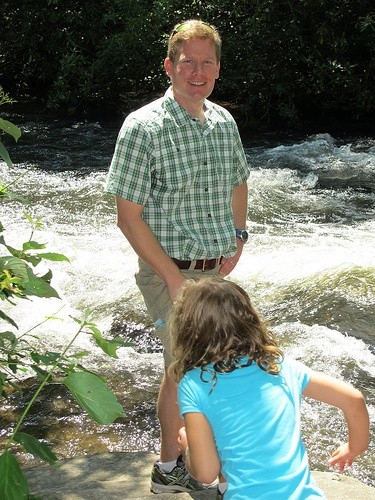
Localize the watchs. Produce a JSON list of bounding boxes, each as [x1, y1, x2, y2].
[[235, 228, 249, 244]]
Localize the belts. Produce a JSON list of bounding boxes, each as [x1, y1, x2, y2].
[[172, 256, 224, 272]]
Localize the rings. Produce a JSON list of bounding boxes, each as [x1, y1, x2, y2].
[[230, 261, 235, 267]]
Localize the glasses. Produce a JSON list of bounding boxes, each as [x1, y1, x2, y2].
[[171, 22, 213, 36]]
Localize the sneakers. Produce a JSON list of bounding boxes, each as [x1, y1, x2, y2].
[[150, 454, 219, 493]]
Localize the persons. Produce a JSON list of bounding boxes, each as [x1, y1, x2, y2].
[[166, 275, 370, 500], [105, 20, 250, 494]]
